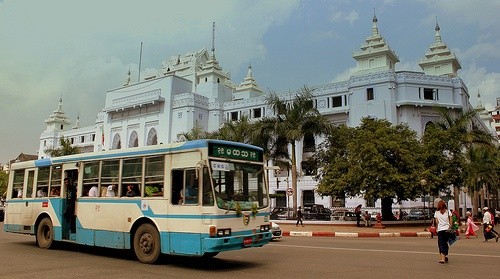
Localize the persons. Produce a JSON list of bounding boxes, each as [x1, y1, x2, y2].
[[355, 205, 362, 227], [106, 184, 116, 196], [37, 183, 60, 198], [144, 178, 163, 196], [89, 179, 98, 197], [295, 206, 304, 226], [478, 206, 482, 218], [126, 184, 136, 196], [17, 189, 23, 197], [465, 213, 479, 239], [428, 210, 438, 239], [64, 178, 77, 233], [179, 179, 198, 204], [482, 207, 500, 243], [362, 211, 371, 226], [465, 209, 470, 218], [394, 208, 408, 221], [450, 209, 461, 240], [434, 199, 453, 264], [204, 169, 221, 198]]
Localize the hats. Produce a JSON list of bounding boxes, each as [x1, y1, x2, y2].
[[483, 207, 488, 210]]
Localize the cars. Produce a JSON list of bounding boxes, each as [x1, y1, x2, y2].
[[394, 210, 427, 221], [272, 207, 298, 220], [271, 221, 283, 240], [304, 203, 378, 221]]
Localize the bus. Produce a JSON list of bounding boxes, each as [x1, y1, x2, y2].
[[3, 139, 274, 262]]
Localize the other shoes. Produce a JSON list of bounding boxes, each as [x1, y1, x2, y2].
[[439, 260, 445, 263], [495, 236, 498, 242], [445, 257, 448, 262]]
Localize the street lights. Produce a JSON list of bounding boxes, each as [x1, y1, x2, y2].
[[463, 188, 467, 217], [420, 179, 428, 231]]
